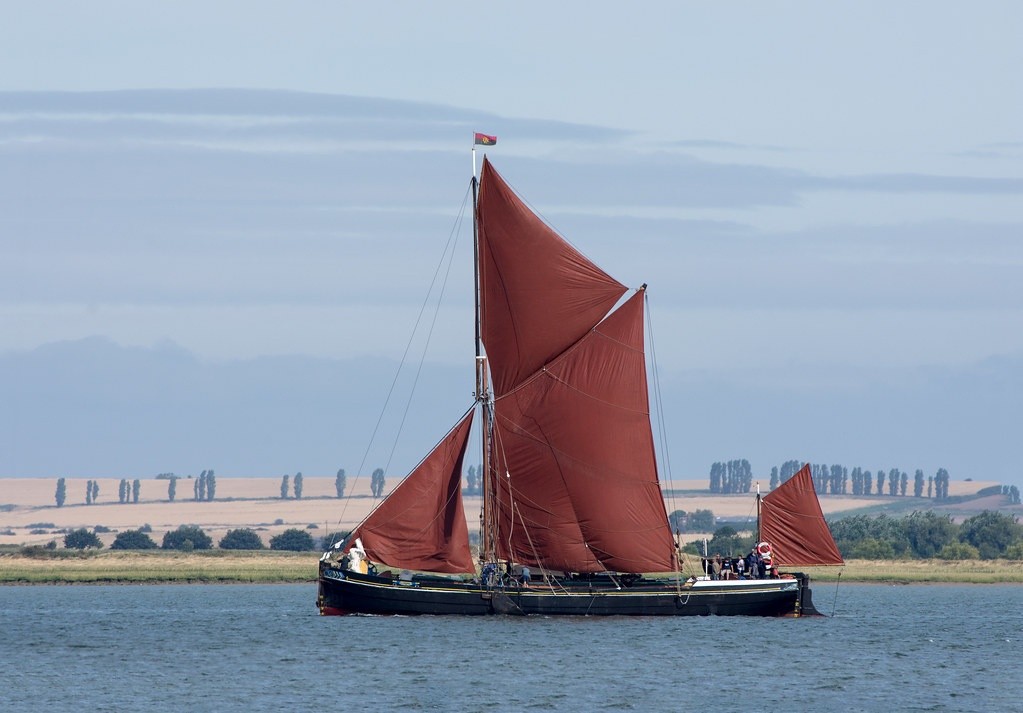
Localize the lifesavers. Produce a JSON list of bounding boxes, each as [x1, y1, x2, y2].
[[757, 541, 772, 556], [759, 555, 773, 570]]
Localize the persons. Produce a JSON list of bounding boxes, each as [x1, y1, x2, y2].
[[712, 548, 780, 581], [497, 562, 512, 586], [482, 567, 496, 585], [337, 555, 350, 570], [519, 566, 531, 588], [619, 574, 635, 588]]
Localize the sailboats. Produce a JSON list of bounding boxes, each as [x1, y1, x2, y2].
[[312, 132, 846, 614]]
